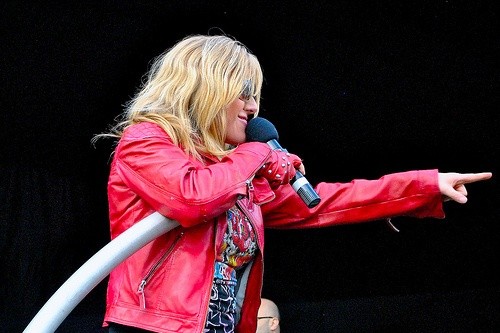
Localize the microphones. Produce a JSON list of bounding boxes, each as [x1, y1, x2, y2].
[[245, 117, 321, 209]]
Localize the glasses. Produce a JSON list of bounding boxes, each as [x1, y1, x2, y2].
[[237, 79, 257, 102]]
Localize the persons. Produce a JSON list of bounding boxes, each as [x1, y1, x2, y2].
[[92, 35, 492, 333], [256, 298, 281, 333]]
